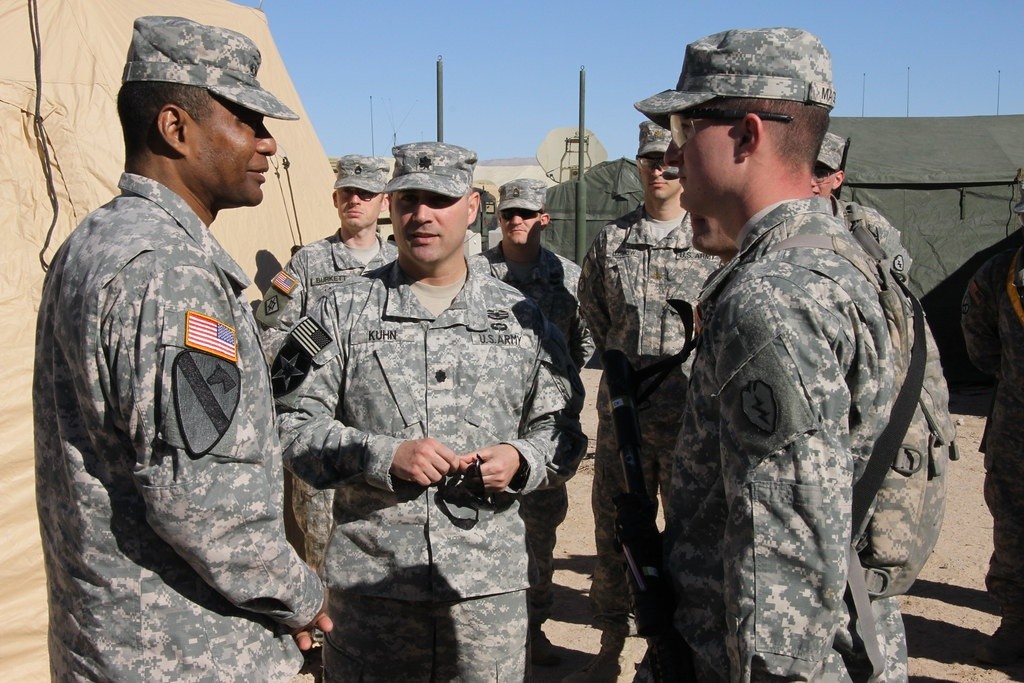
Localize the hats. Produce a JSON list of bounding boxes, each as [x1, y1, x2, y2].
[[1010, 168, 1024, 215], [816, 130, 847, 169], [632, 29, 834, 128], [121, 16, 301, 121], [334, 155, 391, 194], [498, 180, 547, 212], [638, 121, 671, 158], [386, 142, 477, 200]]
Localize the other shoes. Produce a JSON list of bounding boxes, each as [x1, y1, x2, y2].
[[561, 646, 621, 683], [531, 631, 573, 666], [974, 598, 1024, 667], [598, 614, 637, 636]]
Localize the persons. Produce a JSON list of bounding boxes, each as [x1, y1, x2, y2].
[[253, 122, 918, 683], [34, 17, 334, 683], [632, 29, 957, 683], [960, 196, 1024, 672]]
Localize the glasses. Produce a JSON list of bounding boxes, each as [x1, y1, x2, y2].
[[638, 158, 678, 172], [813, 169, 837, 182], [463, 453, 497, 511], [499, 208, 545, 219], [668, 109, 795, 148]]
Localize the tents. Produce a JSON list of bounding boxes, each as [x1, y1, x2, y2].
[[0, 0, 339, 683], [547, 156, 646, 267], [825, 110, 1024, 398]]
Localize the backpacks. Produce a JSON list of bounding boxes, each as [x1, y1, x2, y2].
[[766, 236, 960, 604]]
